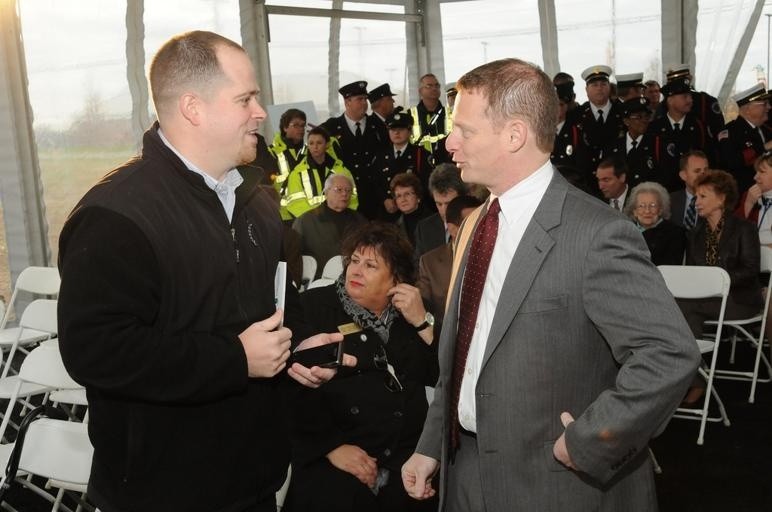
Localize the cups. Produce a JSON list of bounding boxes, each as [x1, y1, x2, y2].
[[373, 341, 404, 393], [329, 186, 353, 194], [291, 123, 307, 129], [425, 84, 441, 88]]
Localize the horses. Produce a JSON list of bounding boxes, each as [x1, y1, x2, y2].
[[284, 341, 344, 374]]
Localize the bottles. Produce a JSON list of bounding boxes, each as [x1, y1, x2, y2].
[[338, 81, 372, 98], [582, 63, 695, 116], [444, 82, 457, 97], [385, 113, 414, 129], [732, 83, 772, 107], [367, 84, 397, 104]]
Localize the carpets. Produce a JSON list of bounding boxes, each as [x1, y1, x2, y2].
[[397, 151, 401, 159], [613, 200, 619, 210], [627, 141, 637, 158], [597, 110, 603, 126], [684, 197, 696, 231], [674, 123, 680, 132], [356, 122, 361, 137], [448, 197, 500, 465]]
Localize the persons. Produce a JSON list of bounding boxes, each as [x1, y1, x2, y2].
[[54, 29, 359, 512], [625, 182, 682, 265], [374, 113, 431, 220], [551, 63, 725, 196], [286, 126, 359, 218], [367, 82, 397, 133], [682, 168, 762, 405], [319, 81, 389, 196], [667, 150, 711, 229], [734, 152, 771, 286], [718, 83, 772, 192], [402, 73, 452, 154], [445, 81, 458, 133], [289, 173, 363, 281], [267, 108, 308, 222], [415, 195, 482, 324], [281, 222, 442, 512], [596, 158, 634, 211], [402, 59, 701, 510], [415, 163, 468, 255], [390, 173, 435, 245]]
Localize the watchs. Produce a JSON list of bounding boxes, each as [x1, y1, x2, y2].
[[416, 312, 434, 332]]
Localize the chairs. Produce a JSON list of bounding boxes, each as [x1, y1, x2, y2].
[[0, 230, 771, 511]]
